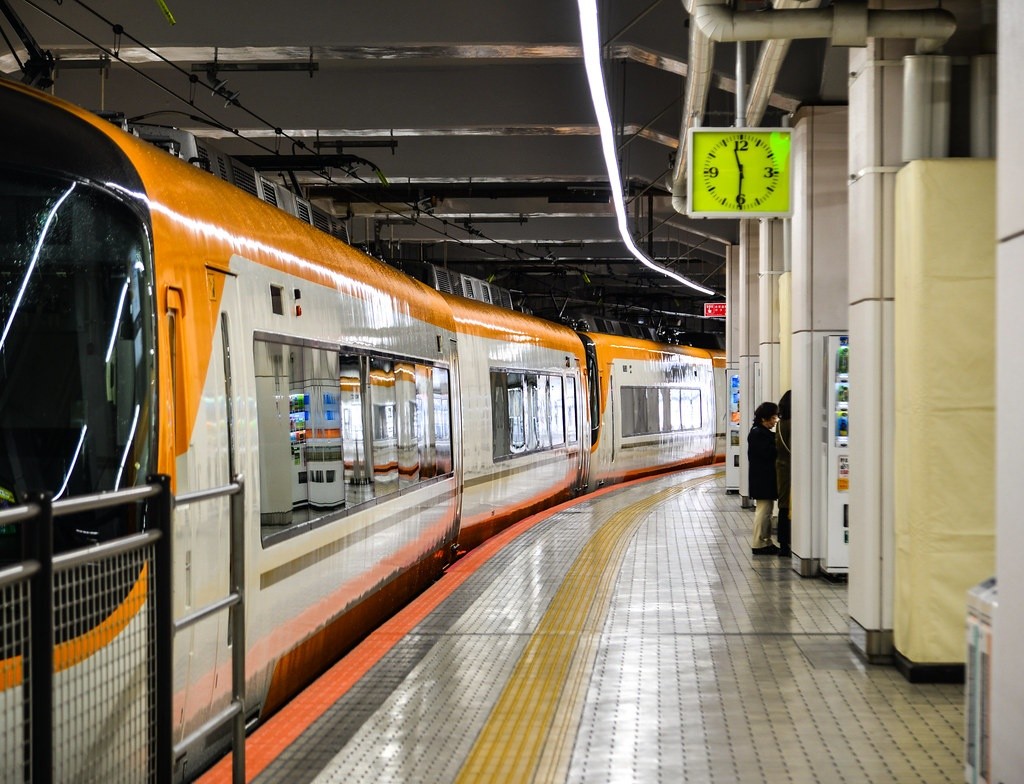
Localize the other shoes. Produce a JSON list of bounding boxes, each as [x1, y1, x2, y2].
[[779, 544, 791, 556], [752, 544, 780, 555]]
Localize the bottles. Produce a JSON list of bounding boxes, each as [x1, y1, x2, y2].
[[839, 347, 849, 373], [839, 457, 850, 478], [289, 394, 304, 414], [839, 419, 848, 446], [290, 415, 303, 431], [838, 385, 848, 402]]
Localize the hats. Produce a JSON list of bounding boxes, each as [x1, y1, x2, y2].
[[754, 402, 778, 417]]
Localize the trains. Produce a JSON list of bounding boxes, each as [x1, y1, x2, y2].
[[1, 78, 726, 784]]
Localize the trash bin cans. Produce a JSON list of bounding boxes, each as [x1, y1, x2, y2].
[[961, 575, 999, 783]]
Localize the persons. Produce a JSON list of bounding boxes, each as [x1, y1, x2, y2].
[[747, 402, 779, 556], [775, 390, 791, 558]]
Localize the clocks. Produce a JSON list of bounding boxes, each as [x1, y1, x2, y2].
[[688, 128, 790, 222]]
